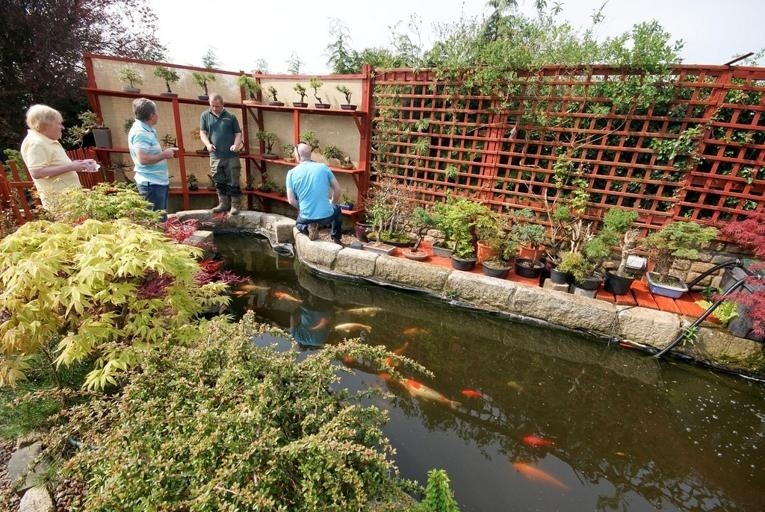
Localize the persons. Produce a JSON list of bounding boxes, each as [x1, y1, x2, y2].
[[199, 93, 247, 213], [19, 104, 98, 211], [286, 142, 343, 246], [128, 98, 179, 222]]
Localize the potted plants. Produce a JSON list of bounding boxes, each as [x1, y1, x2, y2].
[[206, 173, 216, 191], [192, 129, 209, 154], [187, 173, 199, 191], [154, 64, 180, 98], [247, 176, 286, 197], [193, 71, 216, 100], [256, 131, 296, 163], [74, 110, 112, 148], [239, 75, 358, 110], [340, 192, 352, 210], [324, 144, 355, 170], [119, 66, 144, 94], [355, 179, 721, 298]]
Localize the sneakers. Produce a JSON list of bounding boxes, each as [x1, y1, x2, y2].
[[306, 221, 320, 242]]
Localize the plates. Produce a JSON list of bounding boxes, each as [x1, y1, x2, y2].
[[95, 164, 100, 169]]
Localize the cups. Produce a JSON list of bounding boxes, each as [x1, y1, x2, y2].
[[82, 159, 99, 173], [169, 148, 179, 157]]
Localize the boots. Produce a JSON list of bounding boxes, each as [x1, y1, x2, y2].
[[229, 193, 242, 216], [212, 193, 230, 214]]
